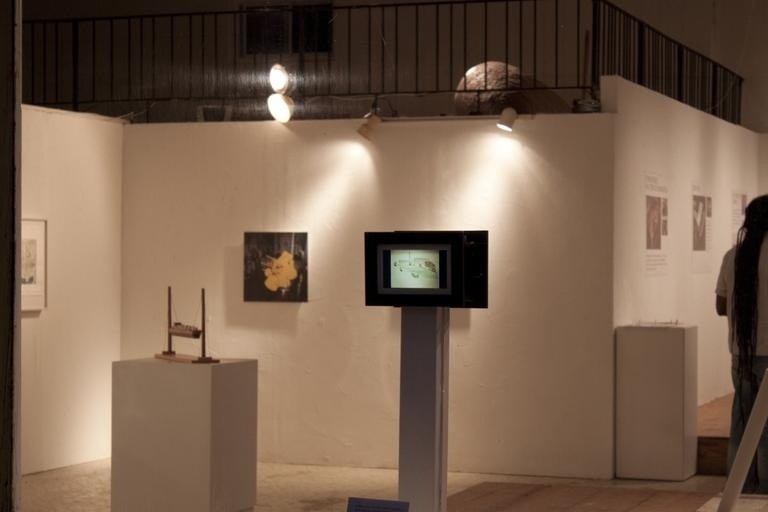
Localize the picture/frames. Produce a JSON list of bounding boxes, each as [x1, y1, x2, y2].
[[21, 218, 47, 313]]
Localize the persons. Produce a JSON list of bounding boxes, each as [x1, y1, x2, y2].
[[715, 191, 766, 492]]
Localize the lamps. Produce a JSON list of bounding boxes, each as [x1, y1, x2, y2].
[[267, 94, 296, 123], [497, 92, 517, 133], [270, 63, 297, 96], [356, 95, 382, 140]]
